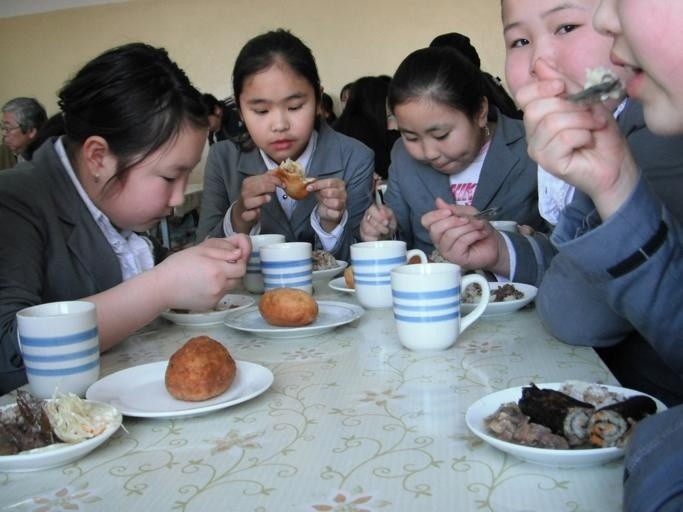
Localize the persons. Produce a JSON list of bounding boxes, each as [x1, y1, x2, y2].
[[416, 1, 683, 409], [0, 29, 561, 397], [513, 1, 683, 512]]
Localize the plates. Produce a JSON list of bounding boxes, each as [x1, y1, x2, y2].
[[457, 281, 539, 318], [326, 276, 356, 294], [225, 300, 363, 339], [156, 291, 254, 327], [82, 357, 274, 422], [0, 396, 124, 474], [464, 379, 667, 468], [312, 259, 348, 281]]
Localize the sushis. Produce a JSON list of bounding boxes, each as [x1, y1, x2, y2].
[[518, 378, 657, 447]]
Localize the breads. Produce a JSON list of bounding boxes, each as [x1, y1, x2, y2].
[[344, 256, 421, 289], [165, 336, 236, 401], [274, 158, 314, 201], [259, 287, 318, 328]]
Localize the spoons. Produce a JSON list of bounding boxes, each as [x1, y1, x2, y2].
[[562, 82, 616, 109], [456, 206, 502, 221]]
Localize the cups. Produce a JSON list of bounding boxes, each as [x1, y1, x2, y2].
[[388, 264, 490, 354], [14, 297, 104, 400], [242, 233, 284, 293], [258, 241, 313, 297], [349, 239, 428, 311], [486, 220, 518, 234]]
